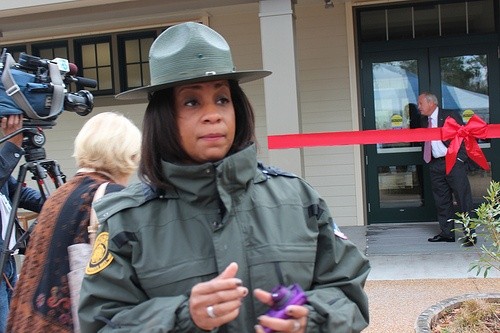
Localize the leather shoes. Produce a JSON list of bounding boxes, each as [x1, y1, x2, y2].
[[428, 234, 455, 242], [465, 236, 477, 246]]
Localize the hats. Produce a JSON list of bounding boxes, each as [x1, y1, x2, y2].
[[116, 21, 273, 99]]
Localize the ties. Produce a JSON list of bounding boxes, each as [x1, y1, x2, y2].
[[422, 118, 432, 163]]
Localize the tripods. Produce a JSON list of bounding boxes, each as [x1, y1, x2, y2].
[[0, 119, 66, 279]]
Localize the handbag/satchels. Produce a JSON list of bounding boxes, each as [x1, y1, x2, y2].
[[66, 181, 108, 333]]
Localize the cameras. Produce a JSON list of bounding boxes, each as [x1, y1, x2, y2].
[[259, 282, 306, 333]]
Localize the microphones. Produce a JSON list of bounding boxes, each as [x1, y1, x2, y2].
[[71, 77, 97, 88], [51, 58, 78, 75]]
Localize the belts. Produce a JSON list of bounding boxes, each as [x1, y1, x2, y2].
[[432, 157, 445, 162]]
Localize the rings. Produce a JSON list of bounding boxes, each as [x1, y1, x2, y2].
[[292, 320, 301, 333], [206, 306, 216, 318]]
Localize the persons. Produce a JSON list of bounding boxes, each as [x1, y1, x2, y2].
[[0, 112, 143, 333], [417, 93, 477, 247], [75, 22, 372, 333]]
